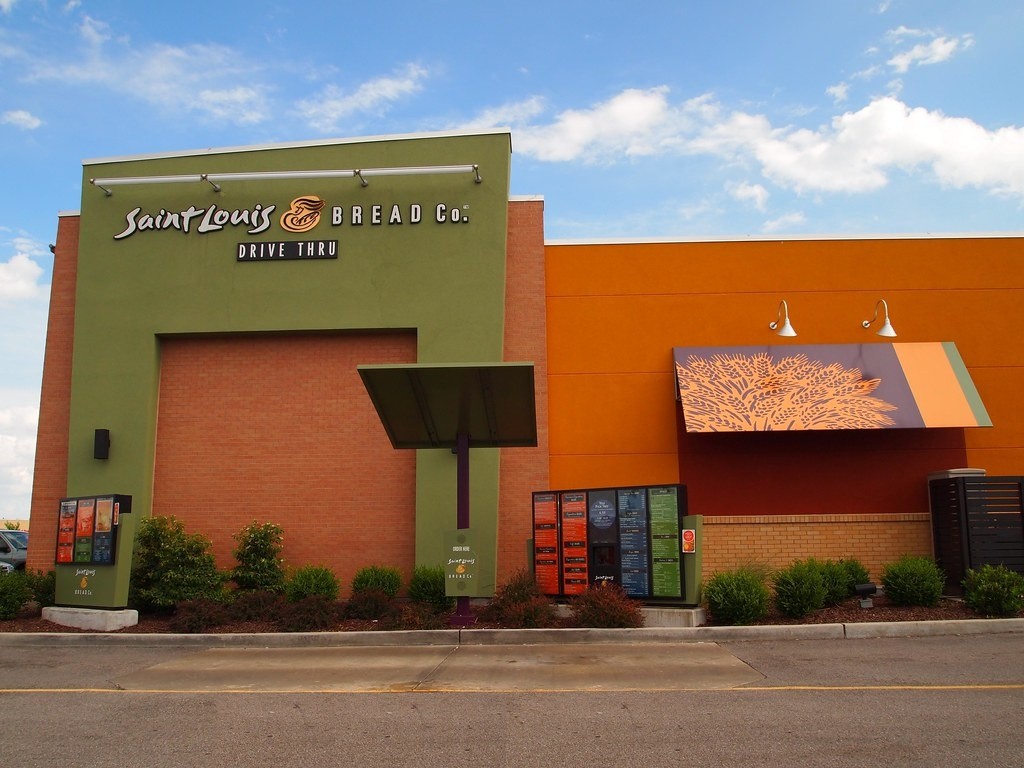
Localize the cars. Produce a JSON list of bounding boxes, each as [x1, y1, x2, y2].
[[0, 530, 28, 573]]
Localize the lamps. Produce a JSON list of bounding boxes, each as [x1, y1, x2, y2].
[[88, 163, 482, 198], [94, 429, 111, 459], [853, 582, 877, 609], [769, 299, 899, 338]]
